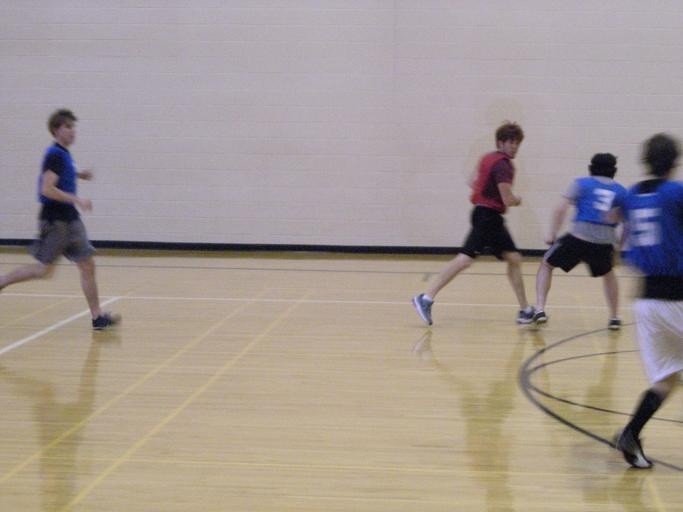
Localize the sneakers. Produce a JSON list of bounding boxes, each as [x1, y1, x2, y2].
[[517, 307, 547, 324], [616, 432, 652, 468], [92, 311, 112, 330], [411, 294, 434, 325], [608, 318, 621, 329]]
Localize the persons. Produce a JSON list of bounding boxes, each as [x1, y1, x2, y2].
[[0, 109, 121, 330], [536, 154, 627, 330], [410, 123, 536, 326], [606, 134, 683, 469]]
